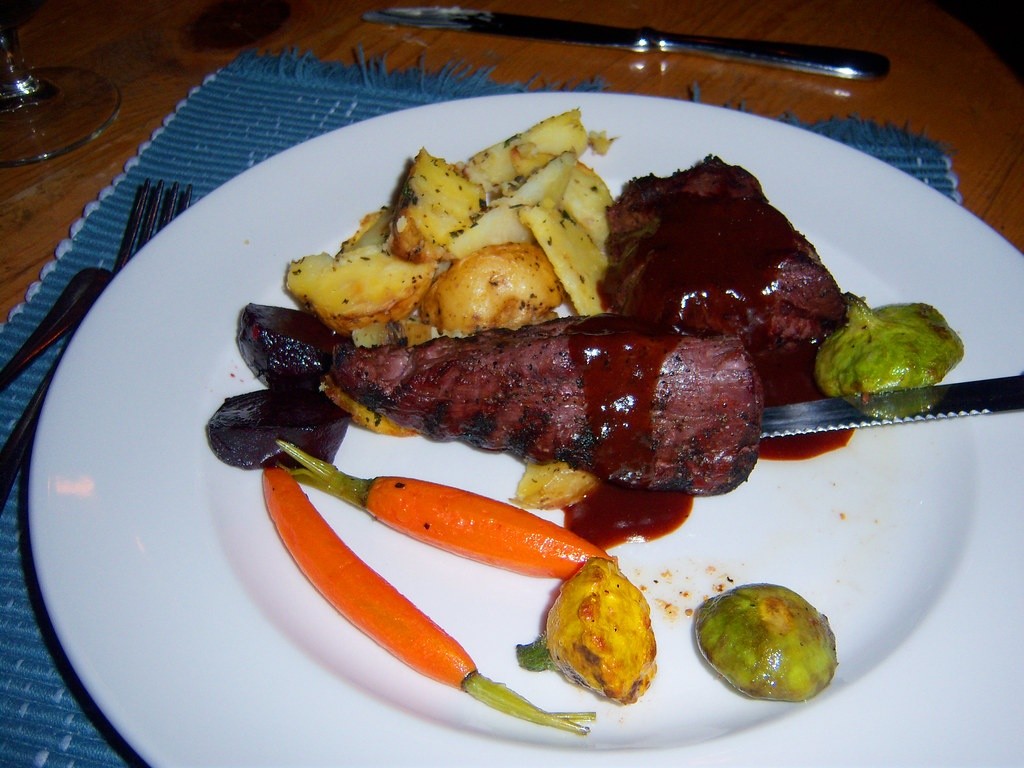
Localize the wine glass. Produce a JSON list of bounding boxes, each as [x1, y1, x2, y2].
[[0, 0, 123, 167]]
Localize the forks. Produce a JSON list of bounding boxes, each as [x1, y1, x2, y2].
[[0, 179, 195, 515]]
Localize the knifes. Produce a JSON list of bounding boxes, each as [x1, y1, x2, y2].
[[359, 6, 892, 79], [760, 371, 1024, 438]]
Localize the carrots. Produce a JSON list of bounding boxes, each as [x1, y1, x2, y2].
[[259, 466, 614, 690]]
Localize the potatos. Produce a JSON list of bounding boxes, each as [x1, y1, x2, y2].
[[287, 110, 617, 437], [510, 457, 601, 511]]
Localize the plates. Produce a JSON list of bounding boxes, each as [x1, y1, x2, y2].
[[28, 91, 1024, 768]]
